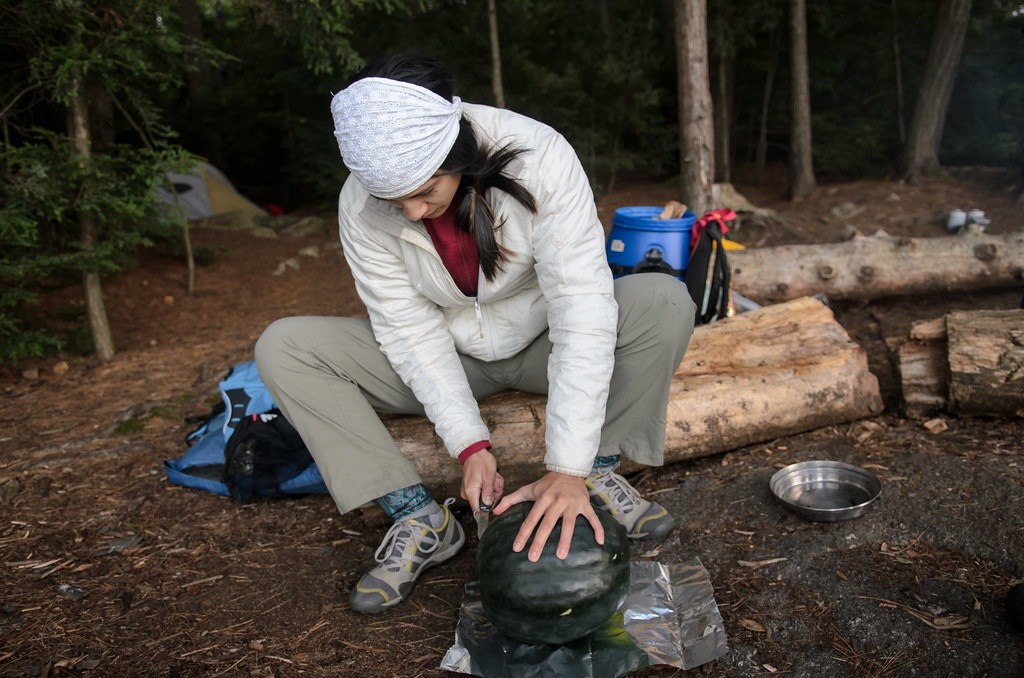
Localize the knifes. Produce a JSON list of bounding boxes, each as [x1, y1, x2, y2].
[[473, 467, 502, 540]]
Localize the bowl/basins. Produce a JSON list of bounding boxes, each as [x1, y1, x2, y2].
[[769, 460, 883, 522]]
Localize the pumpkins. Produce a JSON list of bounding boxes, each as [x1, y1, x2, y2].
[[477, 499, 631, 647]]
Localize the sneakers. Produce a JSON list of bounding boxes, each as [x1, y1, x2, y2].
[[584, 461, 675, 540], [348, 497, 464, 614]]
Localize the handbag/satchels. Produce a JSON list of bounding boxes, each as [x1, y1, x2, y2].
[[163, 360, 330, 497], [220, 408, 313, 502]]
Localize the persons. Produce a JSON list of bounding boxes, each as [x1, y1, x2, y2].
[[254, 77, 697, 614]]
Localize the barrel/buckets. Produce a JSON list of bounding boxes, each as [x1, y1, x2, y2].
[[608, 206, 696, 283]]
